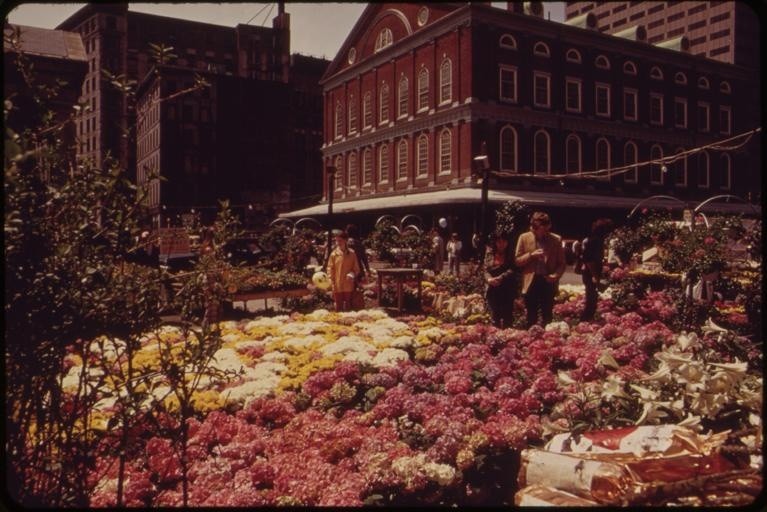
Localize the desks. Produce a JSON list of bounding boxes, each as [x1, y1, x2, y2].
[[233, 288, 311, 312]]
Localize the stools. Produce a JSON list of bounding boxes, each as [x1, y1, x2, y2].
[[377, 268, 422, 309]]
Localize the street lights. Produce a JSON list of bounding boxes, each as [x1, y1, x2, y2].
[[325, 165, 338, 254], [471, 140, 489, 271]]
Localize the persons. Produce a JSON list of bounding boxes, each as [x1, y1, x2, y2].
[[285, 218, 465, 278], [341, 223, 372, 310], [514, 211, 566, 328], [326, 231, 360, 313], [482, 227, 517, 328], [578, 216, 616, 324]]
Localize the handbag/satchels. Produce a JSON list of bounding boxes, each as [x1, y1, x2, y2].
[[351, 289, 364, 309]]
